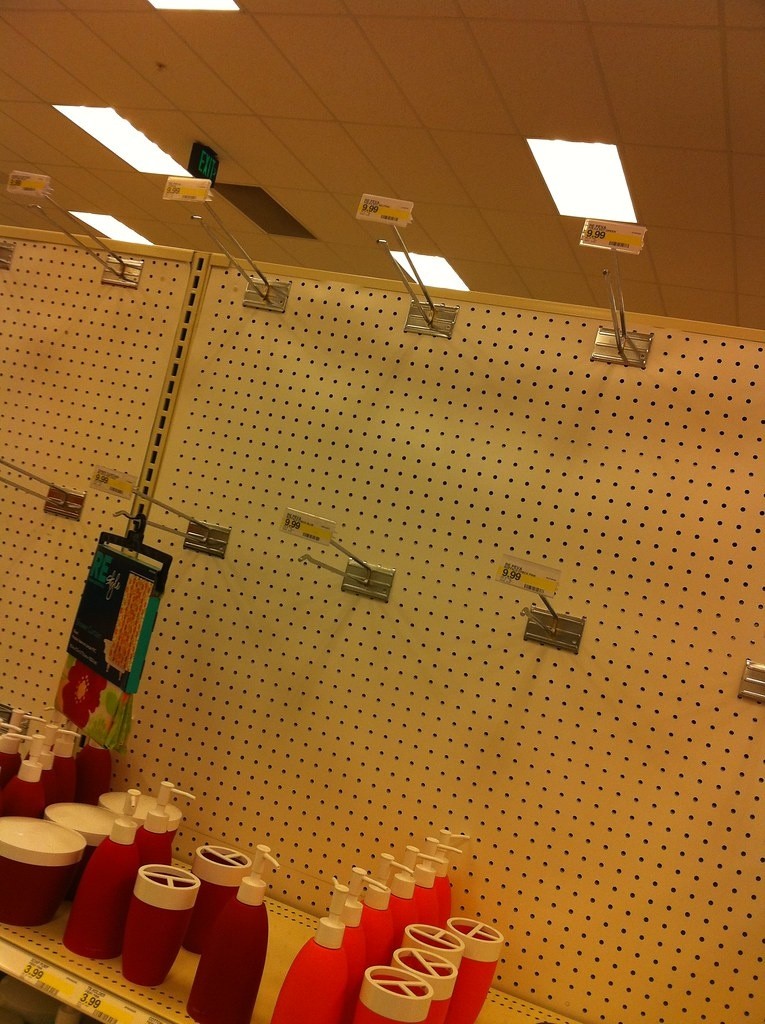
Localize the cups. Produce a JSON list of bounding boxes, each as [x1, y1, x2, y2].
[[98, 792, 181, 845], [0, 816, 87, 927], [44, 803, 120, 899]]
